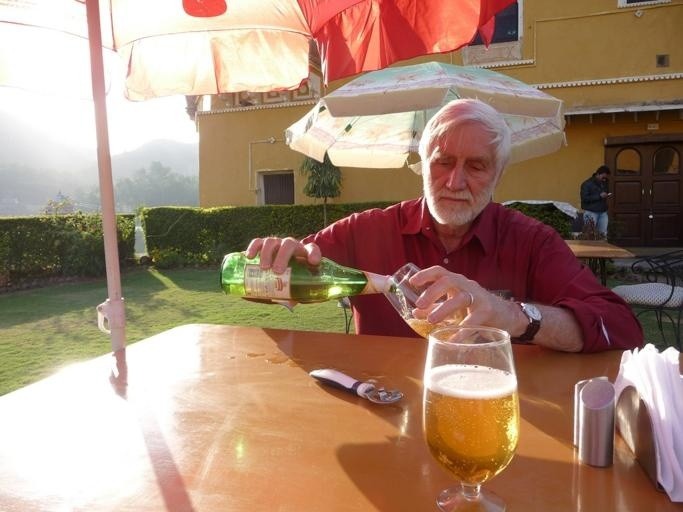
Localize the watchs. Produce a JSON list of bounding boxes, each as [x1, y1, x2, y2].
[[512, 299, 546, 346]]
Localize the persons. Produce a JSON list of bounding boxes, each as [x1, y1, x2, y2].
[[247, 97, 644, 351], [580, 165, 611, 244]]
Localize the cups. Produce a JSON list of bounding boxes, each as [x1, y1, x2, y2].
[[385, 262, 475, 343]]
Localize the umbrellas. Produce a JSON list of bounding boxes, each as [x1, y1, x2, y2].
[[283, 60, 568, 176], [0, 0, 516, 356]]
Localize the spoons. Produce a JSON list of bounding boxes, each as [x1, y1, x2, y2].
[[309, 367, 405, 404]]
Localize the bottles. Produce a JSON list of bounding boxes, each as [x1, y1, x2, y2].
[[216, 250, 396, 304]]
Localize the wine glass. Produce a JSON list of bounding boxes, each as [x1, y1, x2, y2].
[[420, 328, 520, 512]]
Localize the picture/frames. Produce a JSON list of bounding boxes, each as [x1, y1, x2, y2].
[[229, 78, 315, 109]]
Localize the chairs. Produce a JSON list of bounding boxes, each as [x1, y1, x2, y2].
[[609, 249, 682, 354]]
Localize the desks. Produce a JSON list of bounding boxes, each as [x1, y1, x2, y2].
[[0, 317, 683, 512], [558, 239, 634, 288]]
